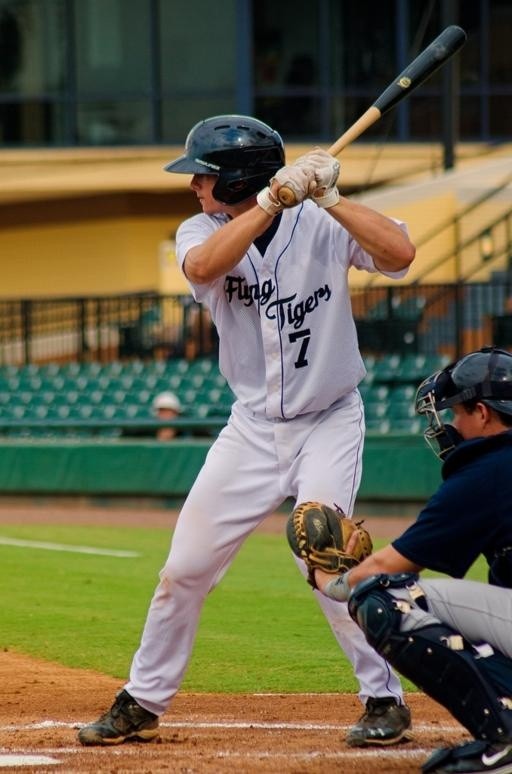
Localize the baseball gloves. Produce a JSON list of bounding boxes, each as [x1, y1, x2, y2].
[[286, 501, 373, 589]]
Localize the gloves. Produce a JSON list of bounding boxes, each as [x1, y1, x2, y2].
[[255, 164, 317, 217], [294, 145, 341, 208]]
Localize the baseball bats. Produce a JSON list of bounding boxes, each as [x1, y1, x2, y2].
[[278, 24, 467, 206]]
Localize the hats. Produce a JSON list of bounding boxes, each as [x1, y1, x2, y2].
[[152, 392, 181, 416]]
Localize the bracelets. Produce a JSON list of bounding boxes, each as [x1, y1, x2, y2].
[[254, 184, 285, 220], [319, 569, 355, 604], [310, 184, 344, 210]]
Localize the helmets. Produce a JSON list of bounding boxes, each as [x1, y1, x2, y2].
[[162, 113, 285, 204], [414, 344, 511, 463]]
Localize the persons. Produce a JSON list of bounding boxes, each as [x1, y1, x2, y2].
[[310, 342, 512, 766], [147, 386, 184, 442], [68, 111, 417, 750]]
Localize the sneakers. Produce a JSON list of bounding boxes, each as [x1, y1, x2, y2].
[[78, 688, 161, 746], [345, 696, 413, 747], [421, 739, 512, 773]]
